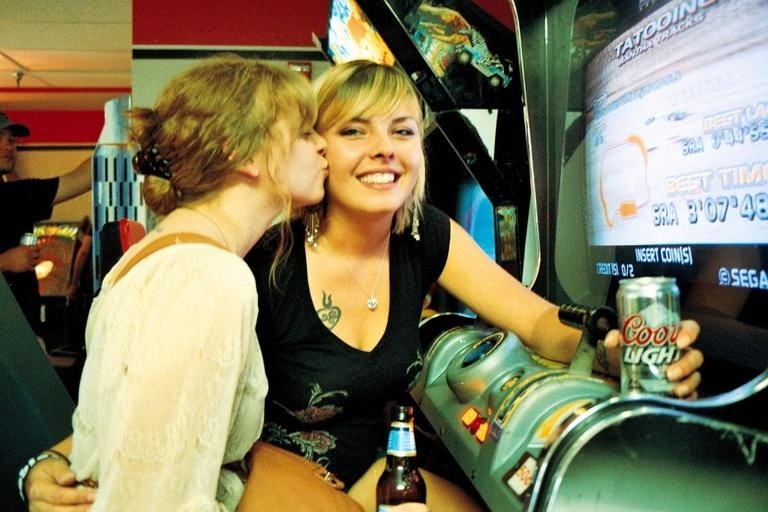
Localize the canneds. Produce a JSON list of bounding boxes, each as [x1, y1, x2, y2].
[[20, 233, 36, 246], [616, 276, 682, 399]]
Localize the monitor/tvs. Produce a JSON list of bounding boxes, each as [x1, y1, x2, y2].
[[451, 175, 497, 272], [576, 0, 768, 400]]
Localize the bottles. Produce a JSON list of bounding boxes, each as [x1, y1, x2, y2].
[[376, 401, 427, 512]]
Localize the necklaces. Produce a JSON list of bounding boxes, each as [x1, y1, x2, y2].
[[319, 227, 393, 310], [179, 203, 229, 248]]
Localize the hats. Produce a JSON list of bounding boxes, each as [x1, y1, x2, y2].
[[0, 112, 29, 137]]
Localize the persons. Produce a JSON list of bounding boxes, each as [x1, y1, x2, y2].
[[11, 55, 706, 512], [67, 51, 331, 512], [0, 110, 95, 339]]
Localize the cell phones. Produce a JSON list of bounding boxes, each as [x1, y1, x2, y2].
[[22, 232, 38, 247]]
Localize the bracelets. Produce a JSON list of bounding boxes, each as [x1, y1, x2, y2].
[[16, 452, 65, 502], [45, 447, 71, 467]]
